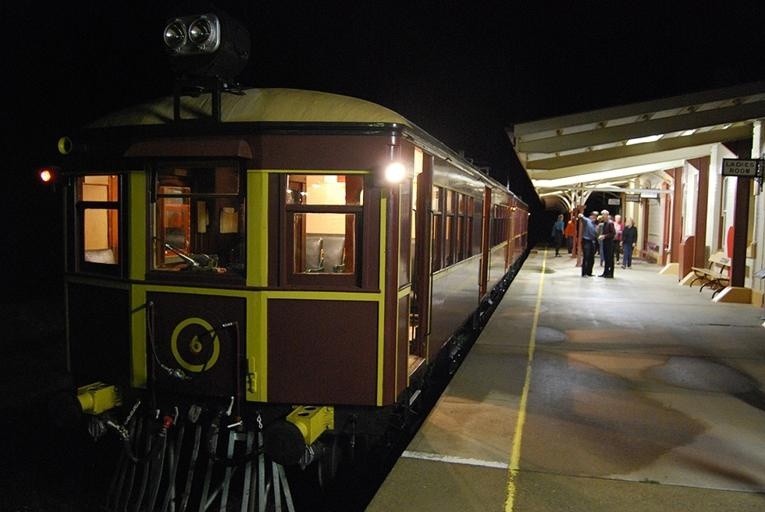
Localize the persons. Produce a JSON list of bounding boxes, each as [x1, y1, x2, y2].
[[551, 207, 638, 279]]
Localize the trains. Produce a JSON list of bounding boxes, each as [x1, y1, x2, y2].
[[40, 87, 531, 496]]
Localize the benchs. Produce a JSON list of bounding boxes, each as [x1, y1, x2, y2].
[[689, 254, 733, 297]]
